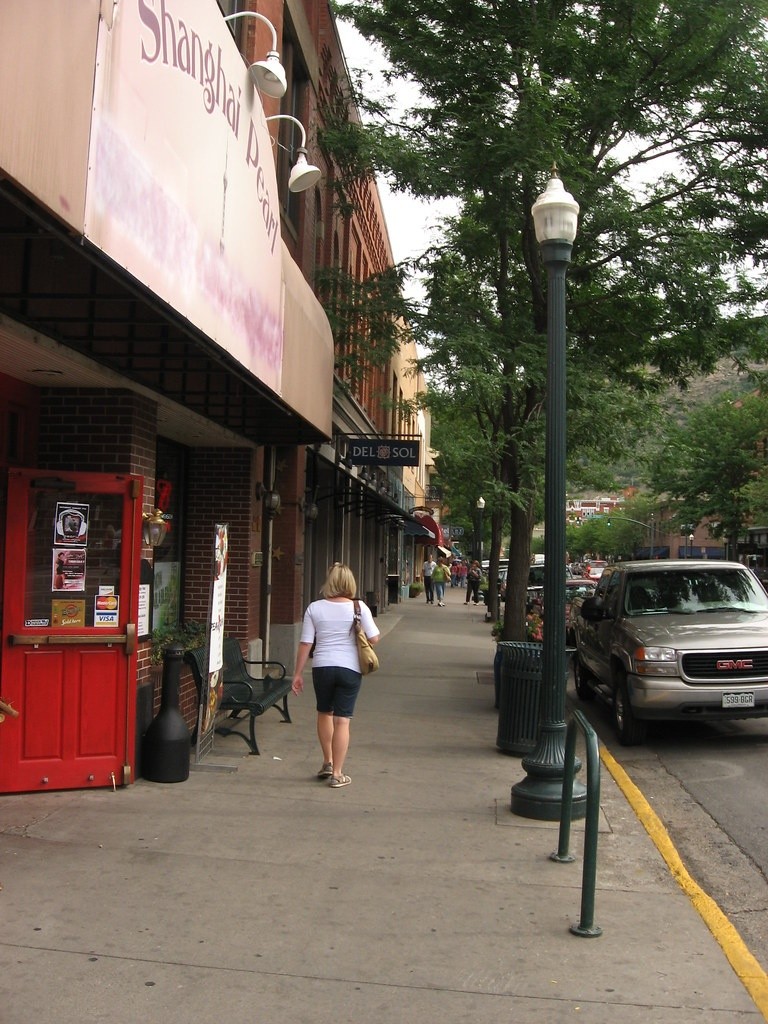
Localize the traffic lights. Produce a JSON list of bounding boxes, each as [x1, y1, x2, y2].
[[607, 519, 611, 527]]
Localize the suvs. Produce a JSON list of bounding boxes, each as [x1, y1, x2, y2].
[[574, 560, 768, 744]]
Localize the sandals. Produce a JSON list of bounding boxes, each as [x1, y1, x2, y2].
[[318, 763, 333, 777], [329, 774, 351, 787]]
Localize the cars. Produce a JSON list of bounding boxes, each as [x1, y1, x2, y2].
[[530, 579, 599, 640], [481, 554, 610, 603]]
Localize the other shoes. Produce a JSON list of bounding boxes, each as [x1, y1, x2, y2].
[[438, 602, 442, 607], [442, 603, 445, 605], [474, 603, 478, 605], [431, 602, 433, 604], [464, 601, 469, 604], [427, 601, 429, 603]]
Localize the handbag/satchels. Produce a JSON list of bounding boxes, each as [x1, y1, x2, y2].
[[443, 565, 451, 583], [348, 599, 379, 675]]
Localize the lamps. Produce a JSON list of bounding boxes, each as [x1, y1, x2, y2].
[[223, 11, 287, 99], [265, 114, 322, 192], [142, 508, 167, 546], [299, 498, 318, 527], [256, 479, 280, 520]]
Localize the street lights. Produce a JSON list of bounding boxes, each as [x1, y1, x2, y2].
[[508, 174, 595, 824], [689, 534, 695, 558], [476, 495, 486, 563]]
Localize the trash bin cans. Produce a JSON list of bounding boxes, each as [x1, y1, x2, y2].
[[496, 641, 577, 756]]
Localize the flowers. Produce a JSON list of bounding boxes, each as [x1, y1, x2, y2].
[[525, 613, 543, 643]]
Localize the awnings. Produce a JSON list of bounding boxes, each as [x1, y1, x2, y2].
[[305, 446, 458, 560]]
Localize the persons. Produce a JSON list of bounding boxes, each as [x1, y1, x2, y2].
[[420, 554, 437, 604], [442, 558, 468, 589], [54, 552, 64, 590], [463, 560, 482, 606], [291, 561, 380, 787], [430, 556, 451, 607]]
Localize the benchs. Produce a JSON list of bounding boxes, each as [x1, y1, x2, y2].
[[185, 635, 292, 755]]
